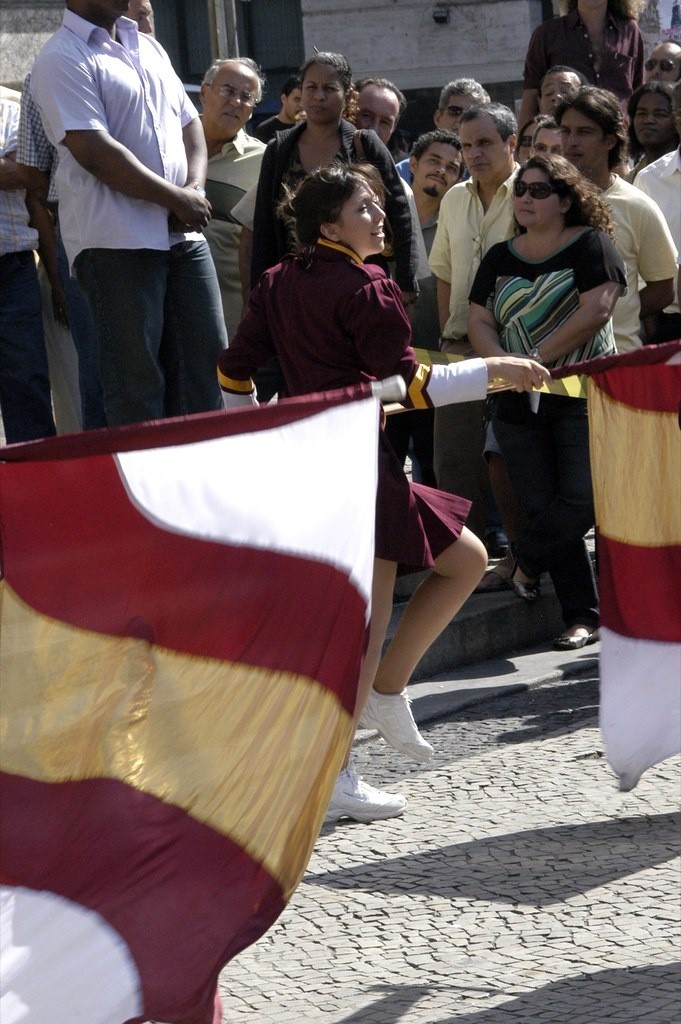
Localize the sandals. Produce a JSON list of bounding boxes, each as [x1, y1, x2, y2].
[[471, 562, 517, 592]]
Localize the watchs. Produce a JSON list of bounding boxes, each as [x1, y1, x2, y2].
[[529, 347, 545, 366]]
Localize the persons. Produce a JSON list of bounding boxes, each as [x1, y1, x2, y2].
[[214, 158, 556, 825], [0, 0, 681, 596], [465, 153, 628, 654]]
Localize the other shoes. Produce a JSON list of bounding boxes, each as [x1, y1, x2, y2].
[[551, 626, 598, 648], [512, 579, 540, 601], [490, 531, 509, 557]]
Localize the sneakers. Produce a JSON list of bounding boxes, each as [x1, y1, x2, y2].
[[323, 759, 406, 821], [360, 688, 435, 761]]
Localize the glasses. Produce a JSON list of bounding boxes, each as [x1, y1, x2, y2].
[[672, 109, 681, 120], [644, 58, 680, 72], [514, 179, 554, 199], [439, 105, 464, 118], [206, 83, 258, 107], [533, 144, 561, 154], [519, 135, 533, 147]]
